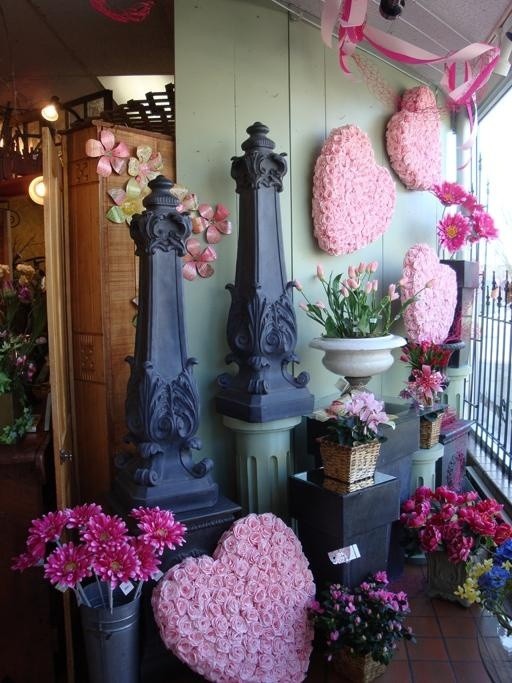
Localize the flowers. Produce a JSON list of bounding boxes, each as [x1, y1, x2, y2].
[[291, 260, 436, 337], [303, 385, 399, 447], [400, 337, 452, 379], [310, 570, 418, 665], [386, 85, 441, 191], [9, 502, 187, 616], [85, 129, 231, 281], [0, 263, 47, 447], [400, 363, 444, 415], [150, 514, 319, 683], [400, 244, 458, 346], [400, 485, 512, 639], [311, 125, 397, 256], [430, 182, 498, 262]]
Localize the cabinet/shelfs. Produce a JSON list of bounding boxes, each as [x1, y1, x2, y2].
[[66, 120, 174, 506]]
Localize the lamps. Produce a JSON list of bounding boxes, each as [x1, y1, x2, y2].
[[491, 31, 512, 77], [378, 0, 405, 21], [41, 94, 85, 128], [28, 175, 48, 205]]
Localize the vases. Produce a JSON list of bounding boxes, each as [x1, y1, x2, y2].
[[308, 334, 407, 397], [494, 614, 512, 655], [439, 260, 480, 369]]
